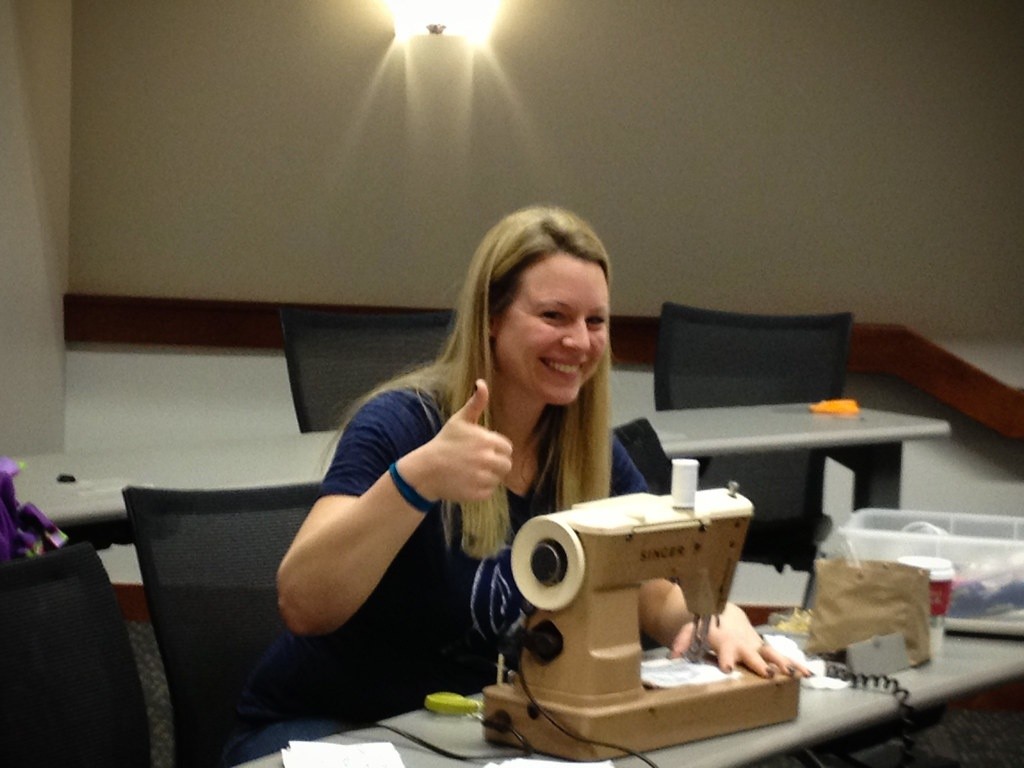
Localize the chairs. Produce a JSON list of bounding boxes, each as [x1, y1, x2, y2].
[[655, 299, 853, 570], [119, 485, 417, 767], [0, 544, 149, 768], [280, 302, 460, 433]]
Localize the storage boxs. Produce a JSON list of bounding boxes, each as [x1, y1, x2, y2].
[[839, 502, 1024, 639]]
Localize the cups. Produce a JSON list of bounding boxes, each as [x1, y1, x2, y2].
[[898, 556, 954, 655]]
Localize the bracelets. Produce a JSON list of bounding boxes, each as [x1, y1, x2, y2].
[[391, 469, 438, 512]]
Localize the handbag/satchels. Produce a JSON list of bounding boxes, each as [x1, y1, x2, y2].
[[802, 558, 930, 667]]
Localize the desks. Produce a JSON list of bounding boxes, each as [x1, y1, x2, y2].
[[233, 610, 1023, 767], [14, 399, 951, 545]]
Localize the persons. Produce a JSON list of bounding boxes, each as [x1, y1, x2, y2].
[[223, 204, 815, 768]]
